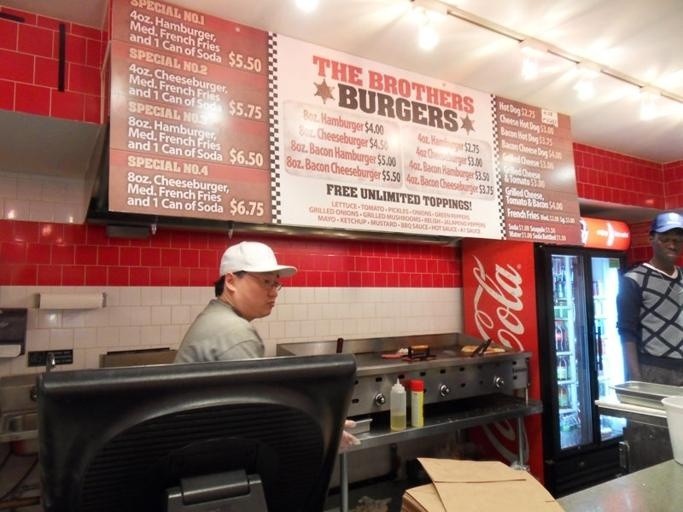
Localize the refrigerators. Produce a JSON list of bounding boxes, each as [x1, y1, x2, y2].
[[462, 215, 635, 493]]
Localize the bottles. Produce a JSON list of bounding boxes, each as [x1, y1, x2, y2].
[[410, 379, 425, 428], [388, 377, 408, 432], [552, 256, 616, 443]]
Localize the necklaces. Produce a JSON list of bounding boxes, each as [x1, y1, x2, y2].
[[649, 258, 683, 295]]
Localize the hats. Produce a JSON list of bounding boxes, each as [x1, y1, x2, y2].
[[219, 241, 297, 277], [651, 212, 683, 233]]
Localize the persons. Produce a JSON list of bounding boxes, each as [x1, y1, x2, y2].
[[172, 240, 360, 452], [615, 211, 683, 473]]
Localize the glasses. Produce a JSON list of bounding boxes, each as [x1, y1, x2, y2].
[[247, 273, 282, 291]]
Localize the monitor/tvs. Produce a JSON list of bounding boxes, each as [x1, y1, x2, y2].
[[37, 354, 357, 512]]
